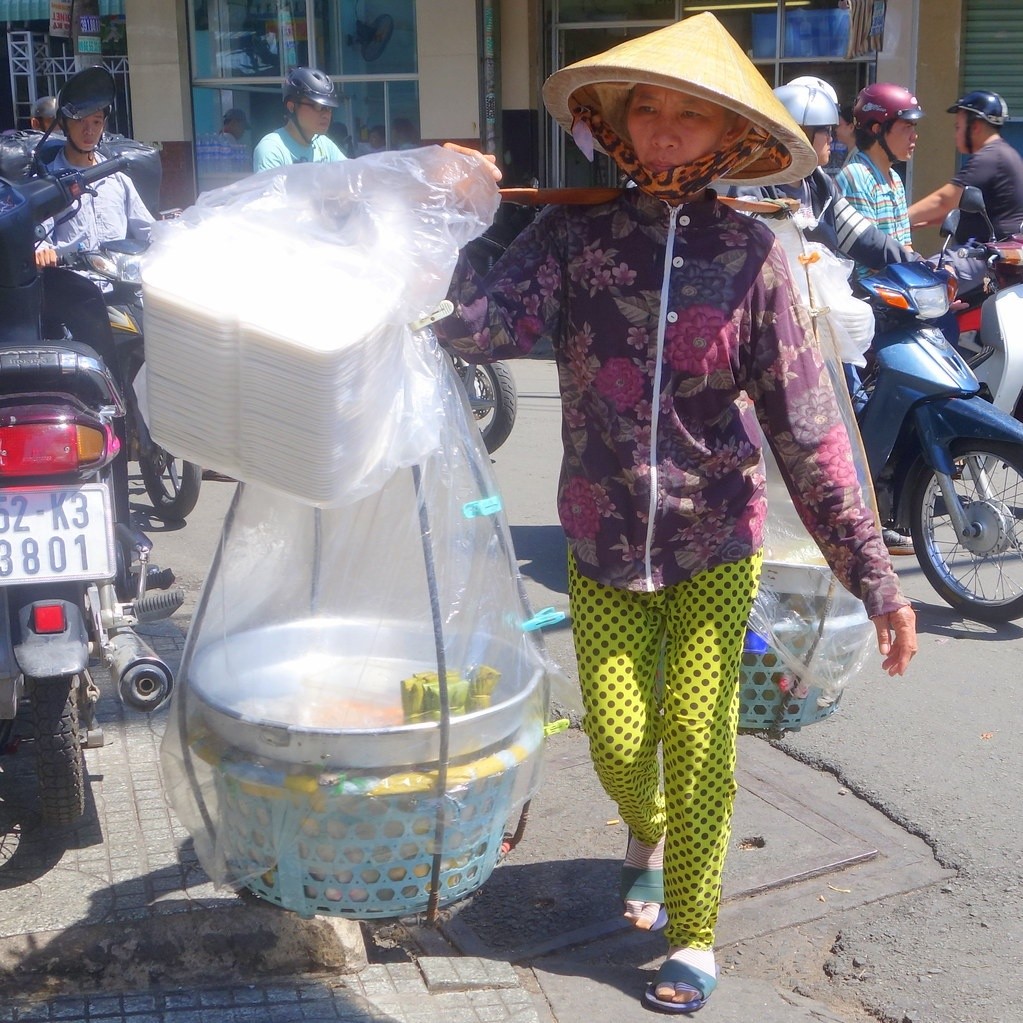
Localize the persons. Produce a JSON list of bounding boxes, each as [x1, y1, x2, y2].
[[727, 74, 1023, 552], [198, 106, 419, 160], [0, 65, 349, 481], [428, 12, 917, 1008]]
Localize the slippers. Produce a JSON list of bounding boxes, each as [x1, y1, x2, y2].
[[645, 948, 713, 1011], [623, 823, 665, 930]]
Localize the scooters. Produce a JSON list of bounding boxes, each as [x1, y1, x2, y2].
[[931, 186, 1023, 479], [0, 60, 190, 850], [850, 204, 1023, 625], [49, 214, 205, 521]]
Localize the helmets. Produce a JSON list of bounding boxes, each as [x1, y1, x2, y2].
[[770, 84, 839, 126], [946, 90, 1007, 125], [54, 87, 111, 123], [283, 67, 338, 107], [853, 82, 926, 128]]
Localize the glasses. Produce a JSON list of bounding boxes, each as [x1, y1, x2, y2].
[[803, 125, 832, 135], [291, 100, 332, 110]]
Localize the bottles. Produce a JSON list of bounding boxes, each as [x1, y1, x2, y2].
[[195, 131, 251, 173]]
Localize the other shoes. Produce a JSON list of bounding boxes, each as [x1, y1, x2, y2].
[[883, 528, 915, 555]]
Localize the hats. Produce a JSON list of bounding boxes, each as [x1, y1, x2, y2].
[[540, 11, 817, 184], [221, 108, 252, 129]]
